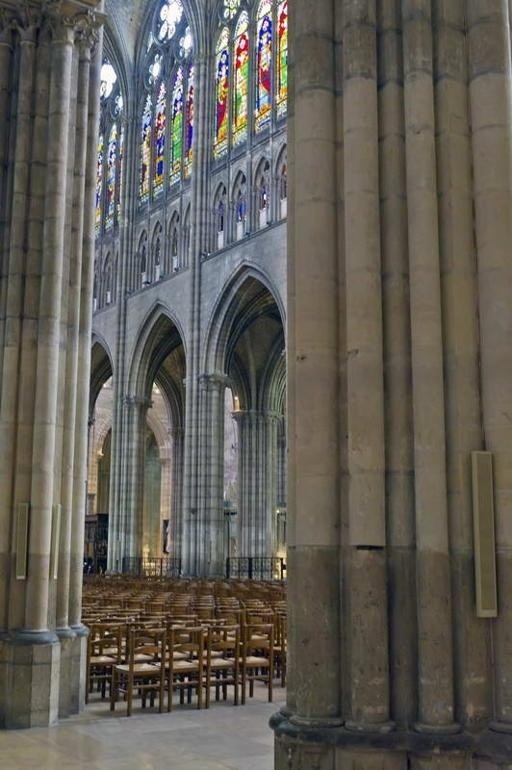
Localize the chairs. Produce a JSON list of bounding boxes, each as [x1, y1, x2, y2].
[[81, 574, 288, 714]]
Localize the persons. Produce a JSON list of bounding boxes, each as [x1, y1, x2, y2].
[[84, 557, 104, 577]]
